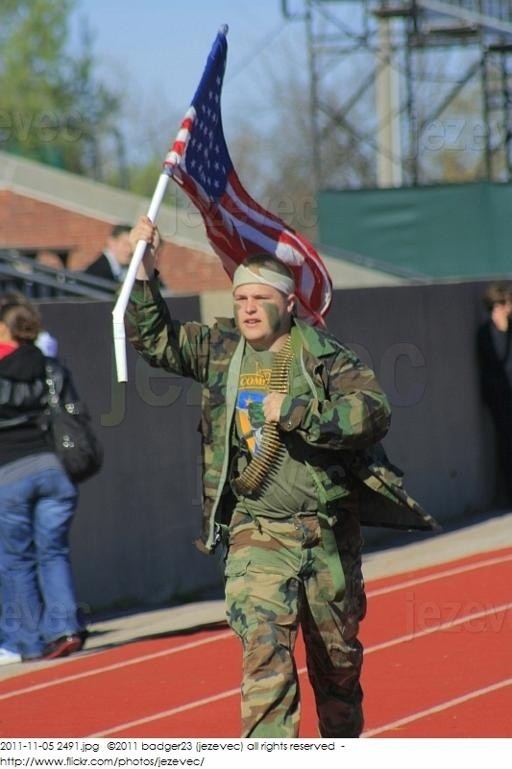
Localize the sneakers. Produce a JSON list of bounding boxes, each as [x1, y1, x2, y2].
[[40, 633, 83, 659]]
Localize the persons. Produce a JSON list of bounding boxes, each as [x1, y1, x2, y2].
[[1, 303, 88, 662], [0, 284, 57, 356], [473, 283, 512, 515], [123, 214, 393, 737], [78, 223, 178, 296]]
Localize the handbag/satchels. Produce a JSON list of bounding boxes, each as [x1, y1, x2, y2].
[[44, 361, 104, 483]]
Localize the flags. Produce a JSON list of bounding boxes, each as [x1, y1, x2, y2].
[[164, 33, 333, 331]]
